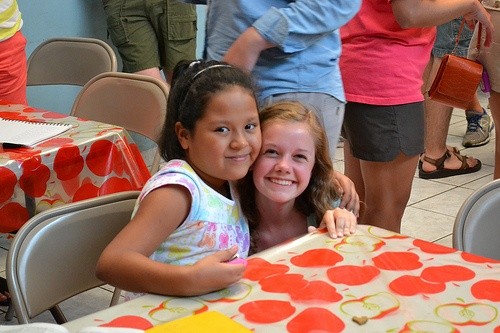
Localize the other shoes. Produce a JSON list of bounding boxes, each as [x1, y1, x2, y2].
[[0, 288, 11, 306]]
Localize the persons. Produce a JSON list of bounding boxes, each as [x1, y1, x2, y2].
[[0, 0, 29, 107], [203, 0, 363, 162], [101, 0, 198, 83], [96, 60, 360, 298], [239, 102, 358, 256], [339, 0, 495, 234], [418, 0, 500, 181]]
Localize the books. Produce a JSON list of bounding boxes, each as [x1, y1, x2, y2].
[[0, 117, 73, 148]]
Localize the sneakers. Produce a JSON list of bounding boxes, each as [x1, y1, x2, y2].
[[462, 107, 494, 148]]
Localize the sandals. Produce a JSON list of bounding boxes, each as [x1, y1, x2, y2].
[[419, 147, 460, 169], [419, 149, 481, 178]]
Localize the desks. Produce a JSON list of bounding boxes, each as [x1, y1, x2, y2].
[[60, 224, 500, 333], [0, 100, 152, 324]]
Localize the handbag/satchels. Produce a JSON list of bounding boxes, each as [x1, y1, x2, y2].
[[428, 17, 484, 109]]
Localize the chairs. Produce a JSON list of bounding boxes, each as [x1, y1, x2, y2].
[[27, 37, 168, 177], [452, 179, 500, 260], [6, 191, 141, 325]]
